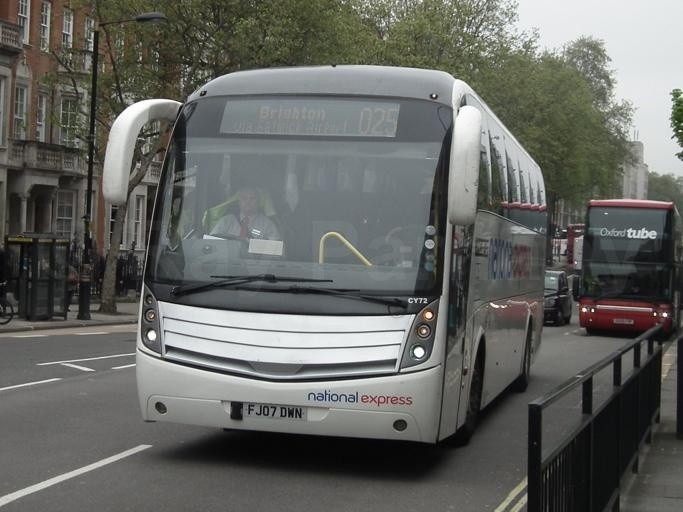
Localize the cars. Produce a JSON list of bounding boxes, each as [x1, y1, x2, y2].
[[540, 269, 572, 324]]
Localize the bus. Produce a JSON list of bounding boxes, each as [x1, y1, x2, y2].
[[93, 61, 552, 455], [565, 196, 682, 337]]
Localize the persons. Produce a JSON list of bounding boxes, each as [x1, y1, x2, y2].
[[207, 183, 280, 245]]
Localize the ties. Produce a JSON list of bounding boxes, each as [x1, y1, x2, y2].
[[240, 214, 249, 237]]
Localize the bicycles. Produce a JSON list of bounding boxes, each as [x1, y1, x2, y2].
[[0, 282, 13, 324]]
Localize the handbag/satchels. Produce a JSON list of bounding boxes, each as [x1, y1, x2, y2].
[[67, 272, 78, 285]]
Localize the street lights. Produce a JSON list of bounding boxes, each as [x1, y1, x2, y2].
[[75, 14, 169, 321]]
